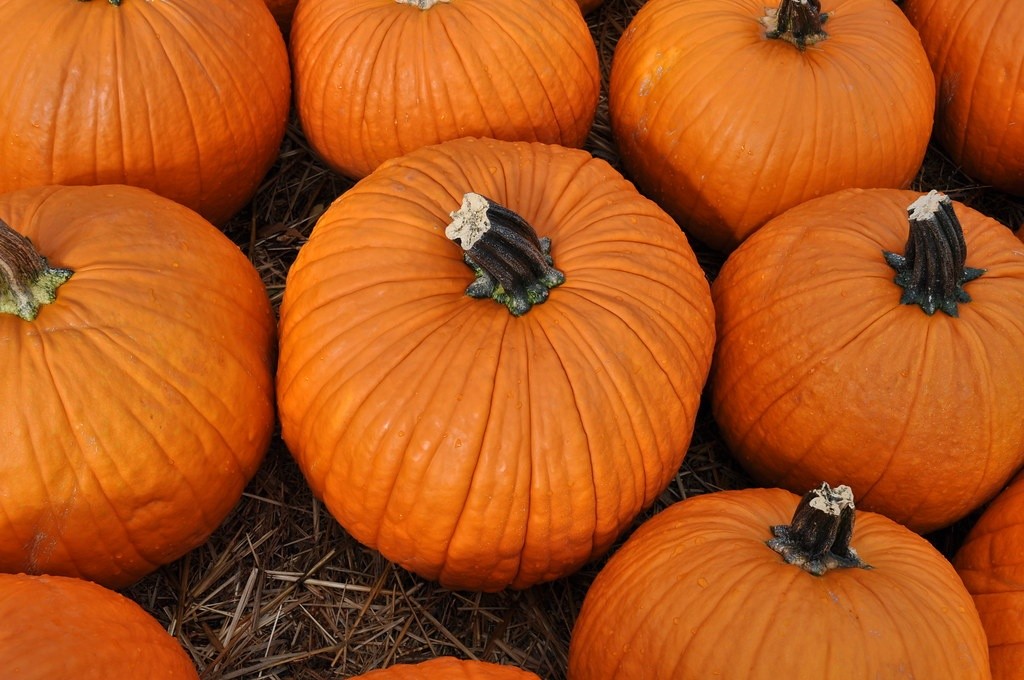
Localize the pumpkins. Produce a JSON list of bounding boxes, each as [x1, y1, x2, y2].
[[0, 0, 1024, 680]]
